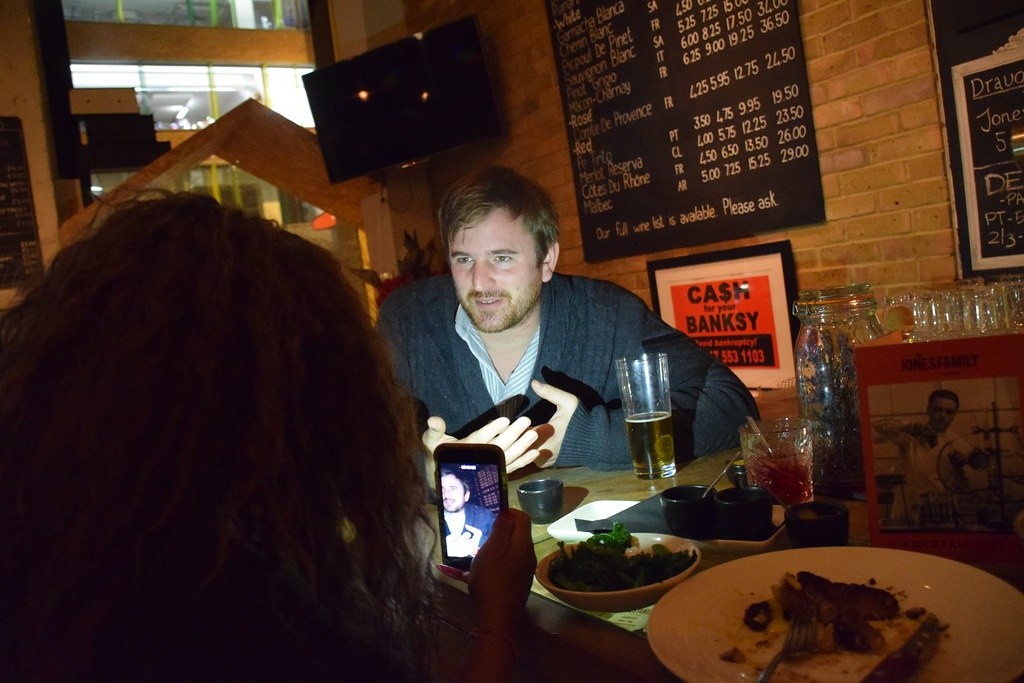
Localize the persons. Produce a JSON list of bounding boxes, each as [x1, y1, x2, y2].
[[440, 467, 496, 557], [872, 389, 974, 529], [0, 188, 445, 683], [375, 168, 762, 503]]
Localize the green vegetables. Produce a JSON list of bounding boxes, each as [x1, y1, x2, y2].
[[543, 521, 699, 591]]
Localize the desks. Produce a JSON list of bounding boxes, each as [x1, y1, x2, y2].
[[392, 439, 1024, 682]]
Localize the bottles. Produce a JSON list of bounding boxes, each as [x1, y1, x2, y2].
[[789, 282, 881, 489]]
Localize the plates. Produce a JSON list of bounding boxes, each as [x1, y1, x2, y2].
[[546, 500, 788, 552], [648, 546, 1024, 683]]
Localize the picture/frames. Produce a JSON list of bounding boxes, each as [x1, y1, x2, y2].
[[645, 240, 801, 392], [952, 28, 1023, 271]]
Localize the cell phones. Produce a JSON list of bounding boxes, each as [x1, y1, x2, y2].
[[433, 443, 509, 571]]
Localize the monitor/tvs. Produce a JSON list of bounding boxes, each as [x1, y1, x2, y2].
[[301, 14, 504, 184]]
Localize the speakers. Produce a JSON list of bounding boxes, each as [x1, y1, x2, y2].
[[56, 113, 158, 179]]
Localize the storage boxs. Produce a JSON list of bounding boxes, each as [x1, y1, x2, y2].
[[854, 324, 1024, 556]]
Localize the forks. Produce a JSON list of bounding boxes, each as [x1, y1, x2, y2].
[[754, 608, 817, 683]]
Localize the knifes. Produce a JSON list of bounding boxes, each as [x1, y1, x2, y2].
[[861, 612, 940, 683]]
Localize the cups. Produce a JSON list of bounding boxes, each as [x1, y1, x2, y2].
[[881, 271, 1023, 344], [713, 487, 775, 541], [660, 485, 716, 540], [785, 502, 850, 549], [739, 418, 813, 504], [614, 353, 677, 479], [725, 456, 747, 486], [518, 478, 564, 525]]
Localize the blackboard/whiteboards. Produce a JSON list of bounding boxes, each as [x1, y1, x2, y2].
[[546, 0, 826, 266], [952, 48, 1023, 271]]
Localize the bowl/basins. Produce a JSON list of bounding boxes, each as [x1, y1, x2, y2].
[[535, 533, 704, 611]]
